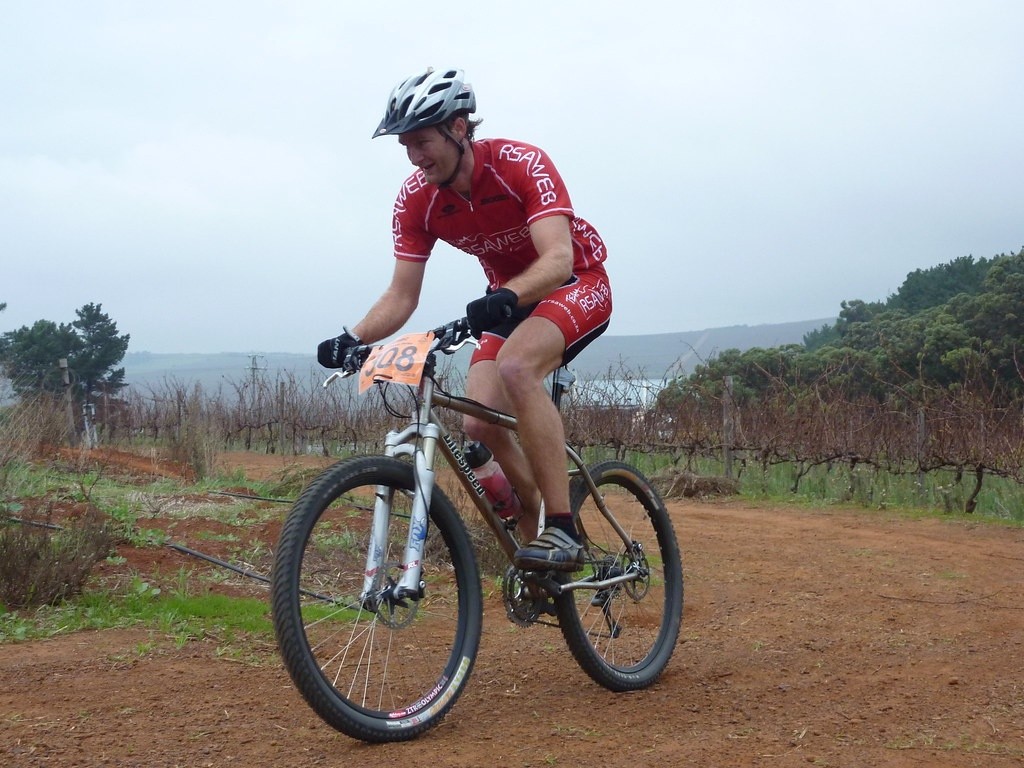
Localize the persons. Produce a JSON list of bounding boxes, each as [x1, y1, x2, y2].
[[317, 64, 612, 572]]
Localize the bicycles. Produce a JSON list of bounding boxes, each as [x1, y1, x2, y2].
[[271, 305, 684, 743]]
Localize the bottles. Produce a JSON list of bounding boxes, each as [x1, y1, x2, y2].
[[463, 440, 525, 522]]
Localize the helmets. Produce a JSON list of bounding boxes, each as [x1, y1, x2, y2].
[[372, 66, 476, 139]]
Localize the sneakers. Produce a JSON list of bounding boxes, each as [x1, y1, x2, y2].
[[506, 585, 547, 624], [514, 527, 586, 572]]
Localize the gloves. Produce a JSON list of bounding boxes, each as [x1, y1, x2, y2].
[[316, 334, 355, 370], [466, 288, 519, 340]]
